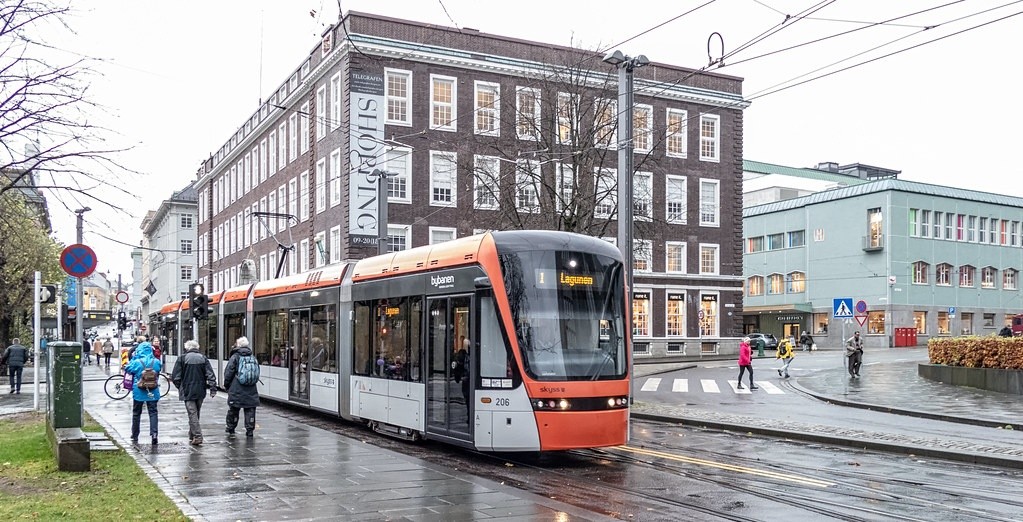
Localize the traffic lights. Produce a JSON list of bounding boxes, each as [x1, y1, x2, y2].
[[117, 312, 127, 331], [189, 283, 206, 321], [202, 295, 214, 320]]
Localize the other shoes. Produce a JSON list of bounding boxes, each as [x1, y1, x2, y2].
[[852, 374, 855, 378], [152, 434, 158, 444], [750, 385, 758, 390], [130, 435, 138, 441], [856, 373, 861, 376], [737, 385, 745, 389], [778, 369, 783, 377], [245, 430, 253, 436], [225, 428, 235, 434], [10, 388, 16, 393], [189, 435, 202, 445], [16, 391, 20, 394], [784, 373, 790, 378]]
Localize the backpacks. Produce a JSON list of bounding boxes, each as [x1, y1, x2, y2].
[[136, 358, 158, 390], [234, 355, 260, 386], [779, 341, 790, 355]]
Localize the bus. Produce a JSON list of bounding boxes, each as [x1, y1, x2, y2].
[[158, 211, 636, 468]]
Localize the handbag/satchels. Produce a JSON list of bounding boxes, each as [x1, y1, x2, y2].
[[123, 372, 133, 391], [811, 343, 817, 350]]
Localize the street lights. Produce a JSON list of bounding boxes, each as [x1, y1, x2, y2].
[[74, 206, 92, 368], [601, 49, 651, 353]]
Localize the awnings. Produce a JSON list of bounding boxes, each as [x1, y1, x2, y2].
[[744, 302, 812, 317]]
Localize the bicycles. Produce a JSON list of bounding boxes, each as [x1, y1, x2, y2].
[[104, 373, 170, 401]]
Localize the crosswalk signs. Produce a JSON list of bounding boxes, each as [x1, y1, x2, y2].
[[832, 297, 855, 319]]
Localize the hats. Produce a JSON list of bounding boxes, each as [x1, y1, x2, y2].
[[743, 337, 752, 343]]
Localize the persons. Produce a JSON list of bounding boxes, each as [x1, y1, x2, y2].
[[736, 336, 758, 389], [102, 337, 115, 366], [94, 338, 102, 365], [171, 339, 217, 445], [775, 335, 795, 378], [222, 336, 260, 435], [0, 337, 29, 395], [82, 339, 92, 364], [127, 335, 162, 370], [90, 334, 96, 345], [805, 332, 815, 352], [845, 331, 864, 378], [1000, 324, 1013, 337], [124, 340, 161, 441], [157, 318, 480, 427], [800, 331, 809, 352]]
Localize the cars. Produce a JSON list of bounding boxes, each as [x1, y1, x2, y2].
[[746, 332, 778, 350]]
